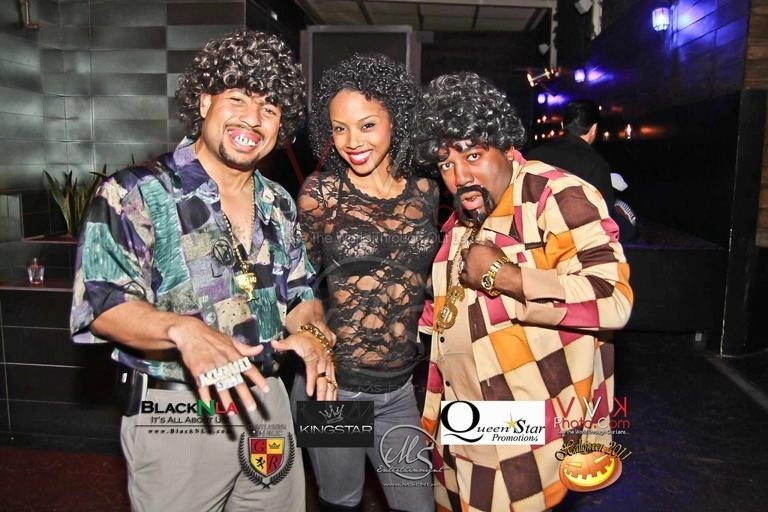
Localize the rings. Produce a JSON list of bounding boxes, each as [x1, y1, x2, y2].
[[331, 380, 339, 390], [316, 370, 333, 384], [197, 356, 252, 392]]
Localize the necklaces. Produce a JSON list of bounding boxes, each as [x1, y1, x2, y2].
[[431, 217, 487, 337], [219, 174, 259, 303]]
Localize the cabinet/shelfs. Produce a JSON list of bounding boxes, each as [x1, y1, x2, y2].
[[0, 287, 123, 456]]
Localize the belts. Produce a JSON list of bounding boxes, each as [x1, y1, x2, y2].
[[147, 354, 285, 394]]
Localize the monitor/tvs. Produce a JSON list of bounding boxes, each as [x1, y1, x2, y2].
[[307, 25, 412, 116]]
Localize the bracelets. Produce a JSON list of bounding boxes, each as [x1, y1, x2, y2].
[[300, 321, 334, 359]]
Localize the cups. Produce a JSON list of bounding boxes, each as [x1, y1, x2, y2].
[[26, 257, 46, 285]]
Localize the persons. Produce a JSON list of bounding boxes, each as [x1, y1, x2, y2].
[[522, 97, 620, 217], [411, 68, 639, 512], [67, 25, 340, 511], [295, 50, 445, 512]]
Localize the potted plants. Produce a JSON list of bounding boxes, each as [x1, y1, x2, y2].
[[20, 153, 134, 282]]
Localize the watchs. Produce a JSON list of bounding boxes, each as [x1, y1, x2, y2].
[[479, 256, 511, 299]]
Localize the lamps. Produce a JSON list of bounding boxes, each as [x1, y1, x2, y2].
[[526, 68, 556, 87], [573, 0, 593, 15], [538, 43, 549, 56], [653, 0, 676, 32]]
